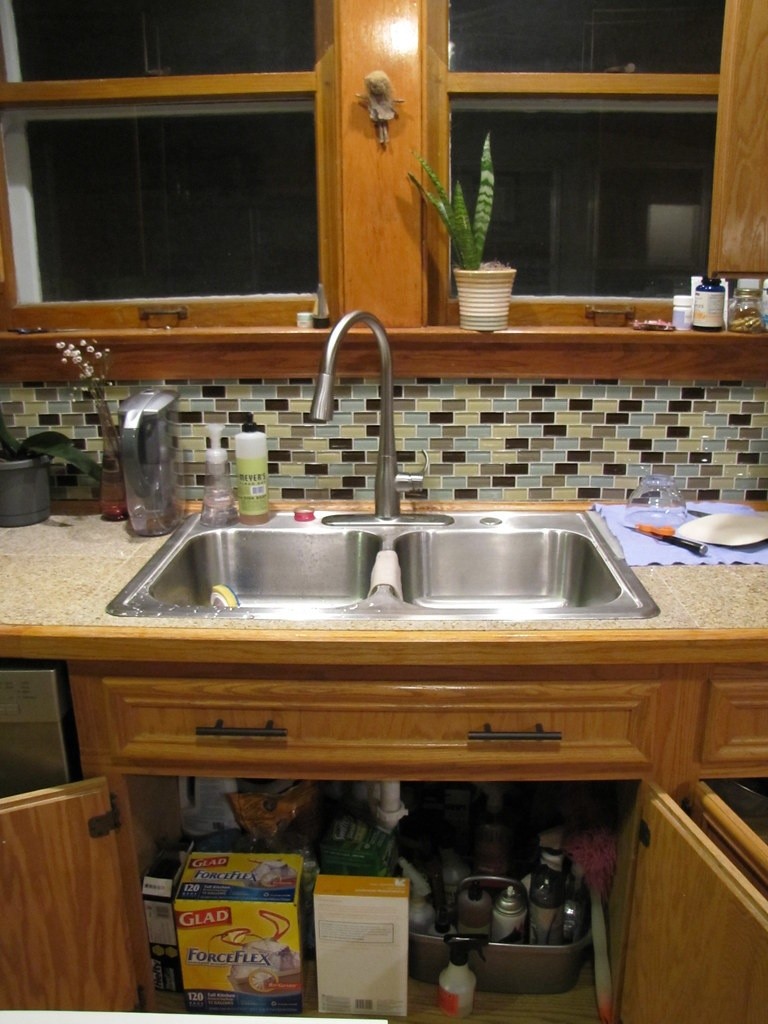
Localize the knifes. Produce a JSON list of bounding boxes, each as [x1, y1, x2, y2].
[[625, 526, 708, 555], [687, 510, 709, 517]]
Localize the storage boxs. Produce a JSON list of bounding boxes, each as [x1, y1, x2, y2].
[[175, 850, 306, 1013], [311, 874, 409, 1016], [142, 839, 194, 993]]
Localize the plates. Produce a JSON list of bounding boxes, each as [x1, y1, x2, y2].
[[678, 513, 768, 546]]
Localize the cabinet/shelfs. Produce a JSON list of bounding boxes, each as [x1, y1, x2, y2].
[[0, 659, 768, 1024]]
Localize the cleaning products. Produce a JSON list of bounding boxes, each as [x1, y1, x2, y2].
[[199, 412, 271, 529], [395, 847, 592, 1017]]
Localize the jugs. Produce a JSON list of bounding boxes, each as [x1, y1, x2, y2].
[[117, 388, 185, 535]]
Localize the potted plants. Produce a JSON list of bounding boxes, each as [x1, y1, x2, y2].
[[403, 131, 517, 331], [0, 409, 102, 529]]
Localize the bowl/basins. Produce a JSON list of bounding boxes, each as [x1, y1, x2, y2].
[[626, 474, 687, 529]]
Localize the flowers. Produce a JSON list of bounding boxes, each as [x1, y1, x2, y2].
[[55, 337, 122, 459]]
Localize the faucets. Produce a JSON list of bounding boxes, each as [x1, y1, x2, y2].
[[308, 309, 429, 519]]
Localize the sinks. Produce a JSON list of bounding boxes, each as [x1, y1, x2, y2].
[[388, 527, 625, 612], [148, 528, 377, 609]]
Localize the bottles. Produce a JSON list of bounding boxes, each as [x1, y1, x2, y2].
[[693, 278, 725, 332], [671, 295, 693, 330], [728, 288, 762, 333], [235, 422, 270, 524], [401, 790, 582, 945]]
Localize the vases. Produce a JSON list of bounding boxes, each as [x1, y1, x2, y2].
[[100, 441, 128, 520]]
